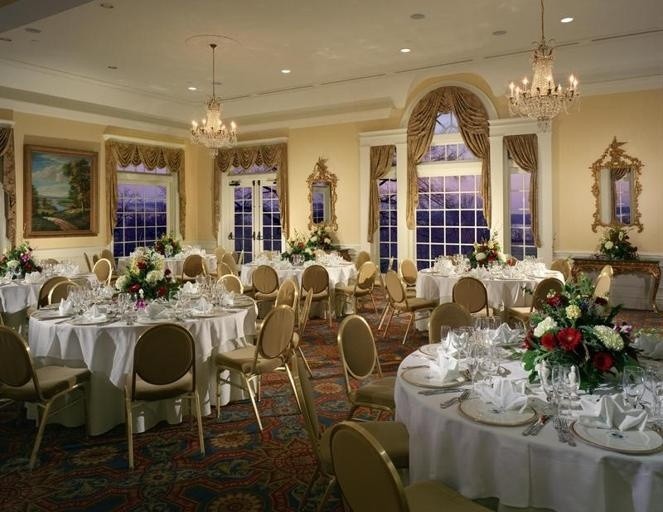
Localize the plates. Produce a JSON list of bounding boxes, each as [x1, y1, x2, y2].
[[403, 344, 663, 454], [28, 293, 249, 326]]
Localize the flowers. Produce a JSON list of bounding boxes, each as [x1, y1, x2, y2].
[[155, 233, 182, 257], [278, 228, 316, 262], [511, 273, 641, 393], [466, 230, 503, 271], [592, 226, 640, 261]]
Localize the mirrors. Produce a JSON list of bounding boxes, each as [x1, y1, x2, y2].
[[304, 156, 338, 231], [587, 135, 644, 235]]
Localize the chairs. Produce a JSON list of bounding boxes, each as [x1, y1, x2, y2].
[[401, 258, 565, 331], [274, 279, 315, 378], [380, 270, 438, 345], [298, 357, 410, 510], [329, 422, 490, 512], [338, 315, 396, 420], [217, 249, 378, 327], [0, 249, 259, 435], [214, 305, 302, 431], [0, 327, 90, 472], [123, 323, 205, 468], [427, 302, 474, 342], [590, 265, 615, 301]]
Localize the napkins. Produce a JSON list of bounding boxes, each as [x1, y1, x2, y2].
[[474, 376, 530, 414], [439, 332, 470, 356], [580, 394, 649, 432], [490, 322, 519, 344], [427, 346, 460, 382]]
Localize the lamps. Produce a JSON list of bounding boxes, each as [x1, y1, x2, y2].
[[187, 43, 239, 160], [504, 0, 583, 134]]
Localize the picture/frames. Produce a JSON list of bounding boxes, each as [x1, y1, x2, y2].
[[22, 142, 100, 237]]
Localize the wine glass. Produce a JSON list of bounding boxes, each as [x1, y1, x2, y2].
[[130, 244, 206, 260], [65, 275, 223, 320], [254, 247, 342, 268], [437, 311, 663, 417], [433, 253, 544, 279], [25, 260, 65, 283]]
